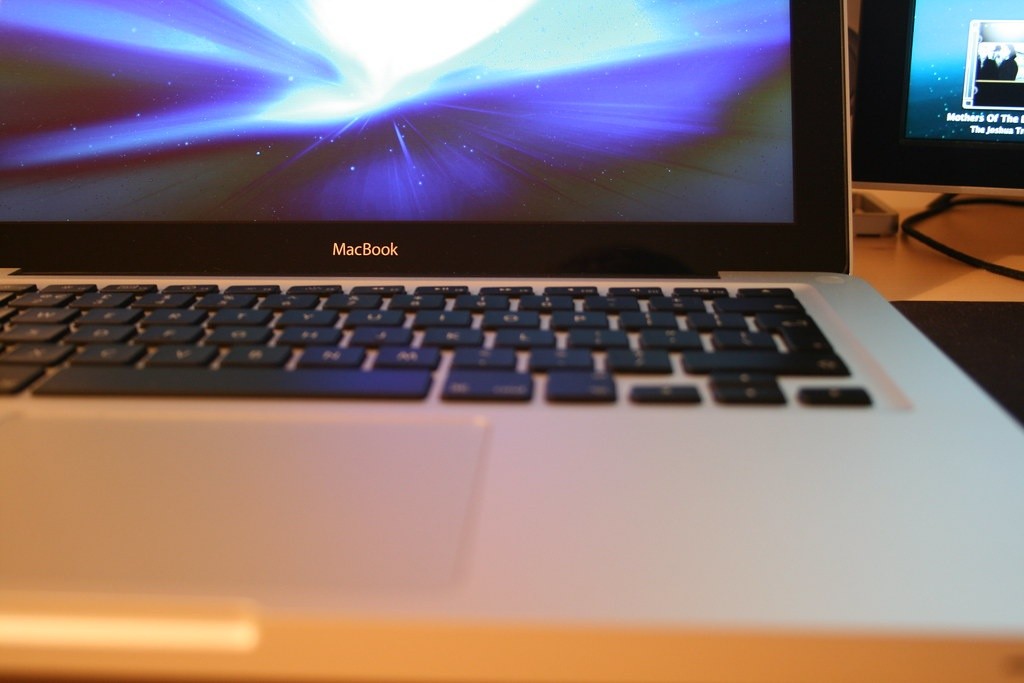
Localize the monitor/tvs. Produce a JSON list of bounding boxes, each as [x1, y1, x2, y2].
[[850, 0, 1024, 198]]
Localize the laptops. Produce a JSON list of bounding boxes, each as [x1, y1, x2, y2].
[[0, 0, 1024, 683]]
